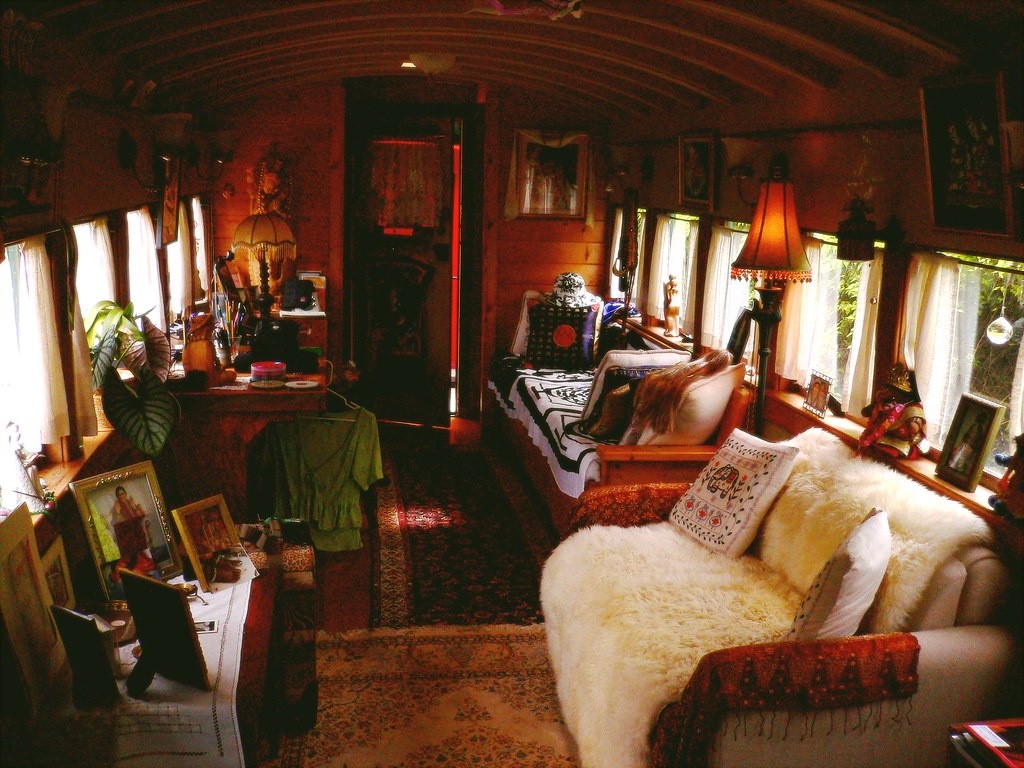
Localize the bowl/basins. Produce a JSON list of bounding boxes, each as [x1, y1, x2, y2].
[[250, 361, 286, 381]]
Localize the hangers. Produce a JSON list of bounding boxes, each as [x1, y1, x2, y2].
[[288, 357, 373, 425]]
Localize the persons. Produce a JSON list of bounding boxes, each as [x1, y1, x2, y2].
[[947, 412, 986, 474], [112, 486, 162, 583], [663, 274, 680, 336], [807, 382, 827, 413]]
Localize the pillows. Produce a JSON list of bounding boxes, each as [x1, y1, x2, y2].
[[523, 302, 599, 368], [664, 427, 802, 555], [620, 349, 732, 446], [584, 360, 683, 430], [581, 347, 695, 422], [638, 360, 747, 445], [779, 503, 900, 643], [510, 290, 606, 362]]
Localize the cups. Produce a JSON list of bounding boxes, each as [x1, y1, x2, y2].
[[229, 335, 242, 363]]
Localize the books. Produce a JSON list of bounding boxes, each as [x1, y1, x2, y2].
[[280, 308, 326, 318]]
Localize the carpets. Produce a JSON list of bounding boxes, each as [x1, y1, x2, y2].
[[365, 434, 554, 627], [249, 616, 583, 768]]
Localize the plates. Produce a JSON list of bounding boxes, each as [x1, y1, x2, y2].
[[285, 380, 319, 389], [251, 380, 285, 388]]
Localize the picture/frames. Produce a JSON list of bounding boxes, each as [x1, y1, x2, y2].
[[40, 535, 78, 610], [914, 66, 1018, 245], [590, 383, 631, 437], [170, 489, 246, 595], [929, 394, 1008, 492], [154, 148, 180, 250], [108, 565, 213, 699], [673, 124, 721, 212], [66, 460, 191, 595], [0, 500, 65, 718], [512, 127, 591, 221], [48, 603, 123, 700]]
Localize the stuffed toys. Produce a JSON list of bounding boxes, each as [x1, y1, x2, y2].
[[234, 317, 319, 375], [988, 433, 1024, 529], [858, 363, 927, 460]]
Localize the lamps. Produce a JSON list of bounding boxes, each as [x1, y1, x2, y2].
[[724, 148, 817, 441], [835, 170, 909, 260], [229, 208, 300, 320]]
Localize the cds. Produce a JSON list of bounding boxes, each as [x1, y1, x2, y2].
[[285, 381, 319, 388], [251, 380, 284, 387]]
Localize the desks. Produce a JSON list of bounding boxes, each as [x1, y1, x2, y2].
[[0, 567, 286, 768], [162, 344, 334, 514]]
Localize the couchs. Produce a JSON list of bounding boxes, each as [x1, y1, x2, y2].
[[536, 427, 1015, 768], [492, 319, 757, 534]]
